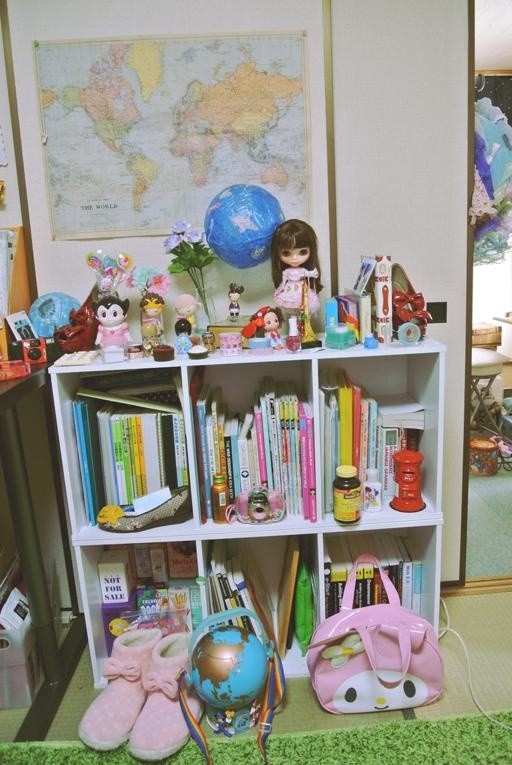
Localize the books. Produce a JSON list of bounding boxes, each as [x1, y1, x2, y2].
[[337, 292, 371, 345]]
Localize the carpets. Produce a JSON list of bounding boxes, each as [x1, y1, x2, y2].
[[1, 712, 512, 764]]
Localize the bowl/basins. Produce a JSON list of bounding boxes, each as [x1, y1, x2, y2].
[[187, 351, 210, 359]]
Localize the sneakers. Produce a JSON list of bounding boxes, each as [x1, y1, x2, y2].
[[97, 486, 192, 533]]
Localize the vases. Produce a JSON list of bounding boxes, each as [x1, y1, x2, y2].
[[191, 285, 219, 326]]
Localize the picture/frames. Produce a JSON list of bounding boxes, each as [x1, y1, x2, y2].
[[0, 0, 342, 337]]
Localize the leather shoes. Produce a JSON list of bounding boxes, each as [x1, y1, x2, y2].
[[55, 281, 100, 352], [391, 263, 433, 335]]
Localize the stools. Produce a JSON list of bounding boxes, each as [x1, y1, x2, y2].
[[468, 346, 511, 451]]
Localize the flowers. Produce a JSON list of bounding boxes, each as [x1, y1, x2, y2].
[[164, 218, 219, 326]]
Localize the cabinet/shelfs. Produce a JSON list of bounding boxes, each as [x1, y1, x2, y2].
[[47, 340, 448, 695]]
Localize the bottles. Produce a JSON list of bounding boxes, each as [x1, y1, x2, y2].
[[331, 465, 362, 525], [203, 330, 217, 354], [362, 465, 383, 515], [212, 474, 231, 523], [285, 316, 304, 353]]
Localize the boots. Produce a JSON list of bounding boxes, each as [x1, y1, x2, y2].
[[77, 624, 163, 751], [127, 630, 205, 761]]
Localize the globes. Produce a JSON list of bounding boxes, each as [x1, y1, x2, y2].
[[184, 608, 274, 731]]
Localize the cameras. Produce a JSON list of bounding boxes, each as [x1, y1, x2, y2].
[[9, 337, 61, 366]]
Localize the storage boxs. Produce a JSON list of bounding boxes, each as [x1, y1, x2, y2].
[[498, 414, 511, 440], [1, 595, 46, 713], [472, 371, 504, 409], [200, 313, 259, 348]]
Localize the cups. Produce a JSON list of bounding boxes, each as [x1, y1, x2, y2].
[[155, 348, 174, 362], [220, 332, 243, 356]]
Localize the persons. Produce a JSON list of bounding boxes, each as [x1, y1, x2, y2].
[[86, 219, 319, 361]]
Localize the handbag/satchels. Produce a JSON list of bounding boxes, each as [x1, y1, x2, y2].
[[306, 553, 447, 716]]
